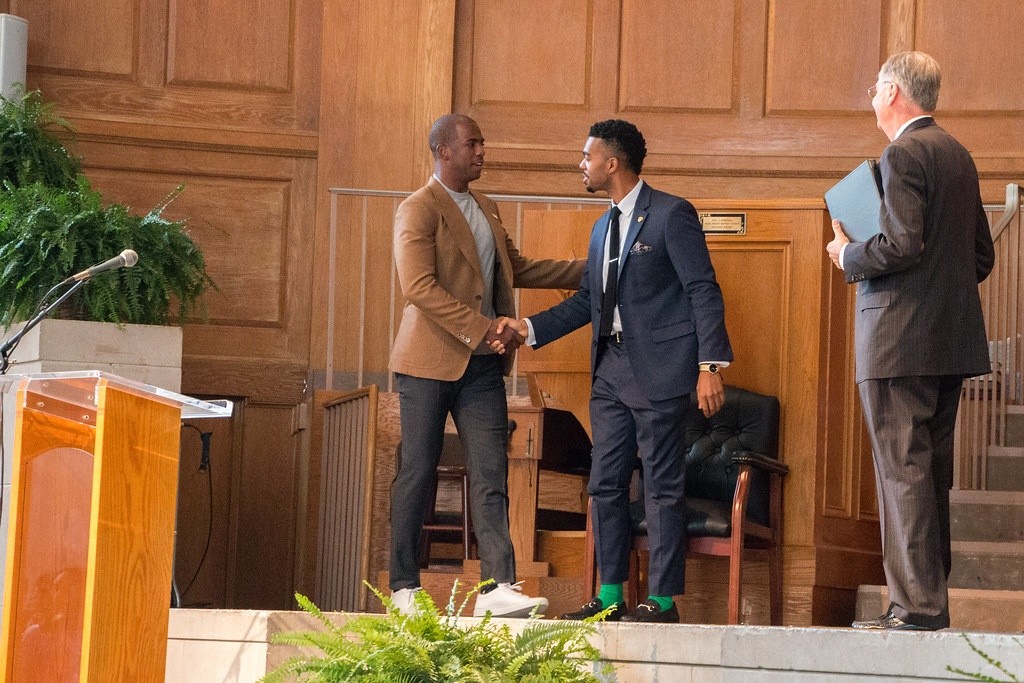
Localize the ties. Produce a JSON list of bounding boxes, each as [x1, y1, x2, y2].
[[599, 207, 622, 337]]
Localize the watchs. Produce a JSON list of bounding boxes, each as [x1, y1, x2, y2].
[[699, 364, 721, 374]]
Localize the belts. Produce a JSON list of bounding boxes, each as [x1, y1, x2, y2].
[[608, 331, 626, 343]]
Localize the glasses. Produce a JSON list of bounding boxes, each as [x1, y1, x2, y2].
[[867, 81, 890, 100]]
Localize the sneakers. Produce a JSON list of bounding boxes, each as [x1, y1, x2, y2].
[[473, 580, 549, 617], [384, 587, 436, 617]]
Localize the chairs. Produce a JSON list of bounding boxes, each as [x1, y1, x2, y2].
[[585, 385, 792, 626], [395, 419, 517, 570]]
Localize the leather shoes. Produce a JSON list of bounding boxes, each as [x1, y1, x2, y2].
[[852, 610, 936, 630], [619, 599, 680, 623], [560, 596, 627, 621]]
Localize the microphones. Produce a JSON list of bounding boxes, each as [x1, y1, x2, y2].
[[64, 249, 138, 285]]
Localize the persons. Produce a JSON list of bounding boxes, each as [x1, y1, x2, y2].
[[826, 48, 998, 634], [491, 118, 733, 624], [386, 114, 594, 617]]
